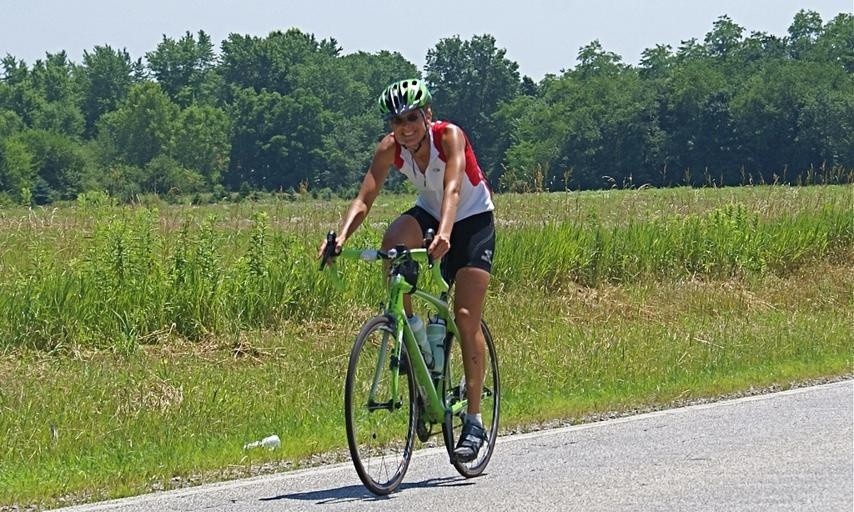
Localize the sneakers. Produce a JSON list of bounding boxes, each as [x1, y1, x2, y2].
[[450, 423, 487, 464]]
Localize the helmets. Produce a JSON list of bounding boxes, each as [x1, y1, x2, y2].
[[378, 79, 433, 122]]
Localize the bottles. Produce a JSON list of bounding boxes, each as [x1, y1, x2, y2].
[[406, 313, 447, 374]]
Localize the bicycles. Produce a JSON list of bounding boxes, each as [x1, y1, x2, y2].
[[317, 229, 501, 496]]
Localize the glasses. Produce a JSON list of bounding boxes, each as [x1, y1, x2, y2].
[[390, 114, 424, 125]]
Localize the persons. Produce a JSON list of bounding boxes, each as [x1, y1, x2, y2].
[[311, 79, 496, 462]]
